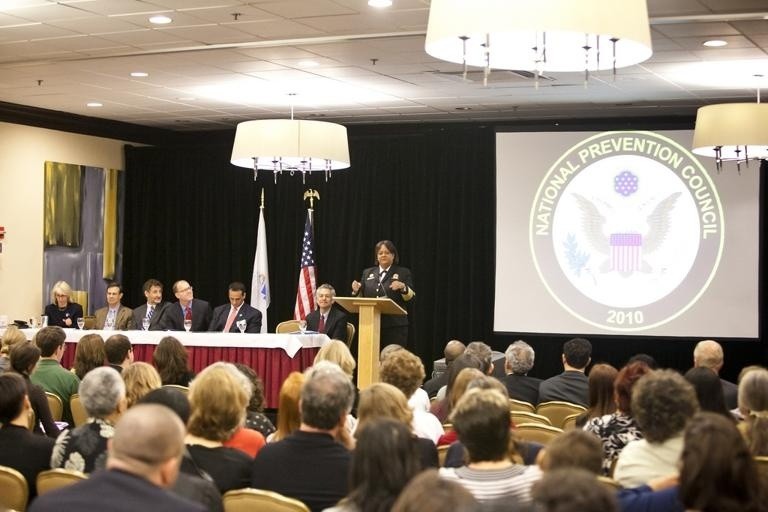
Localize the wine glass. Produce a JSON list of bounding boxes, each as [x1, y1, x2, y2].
[[77, 318, 84, 330], [184, 319, 192, 331], [299, 320, 307, 336], [238, 323, 247, 333], [142, 318, 151, 331], [105, 317, 114, 330]]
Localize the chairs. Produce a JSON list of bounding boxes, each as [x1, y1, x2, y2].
[[752, 455, 768, 486], [429, 395, 625, 492], [276, 320, 306, 333], [344, 321, 355, 347]]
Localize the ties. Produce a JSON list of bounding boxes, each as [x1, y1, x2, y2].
[[147, 306, 155, 320], [318, 315, 327, 333], [185, 305, 193, 320], [223, 307, 237, 332]]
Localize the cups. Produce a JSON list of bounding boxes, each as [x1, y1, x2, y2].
[[0, 315, 9, 330], [30, 319, 39, 330], [40, 316, 49, 329]]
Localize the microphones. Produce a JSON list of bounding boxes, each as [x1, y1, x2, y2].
[[372, 271, 389, 299]]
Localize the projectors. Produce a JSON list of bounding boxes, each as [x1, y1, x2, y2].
[[433, 349, 506, 377]]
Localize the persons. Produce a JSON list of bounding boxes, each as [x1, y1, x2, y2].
[[0, 321, 767, 511], [131, 278, 175, 331], [207, 281, 262, 333], [162, 280, 212, 331], [351, 239, 415, 351], [43, 280, 84, 328], [306, 282, 347, 344], [93, 280, 135, 330]]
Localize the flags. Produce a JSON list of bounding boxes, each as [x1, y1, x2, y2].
[[292, 208, 318, 320], [250, 206, 270, 333]]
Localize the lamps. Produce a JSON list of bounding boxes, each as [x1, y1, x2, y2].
[[230, 93, 352, 185], [691, 73, 768, 177], [424, 0, 654, 93]]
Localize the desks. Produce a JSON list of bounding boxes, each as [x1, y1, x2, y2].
[[0, 326, 328, 413]]
